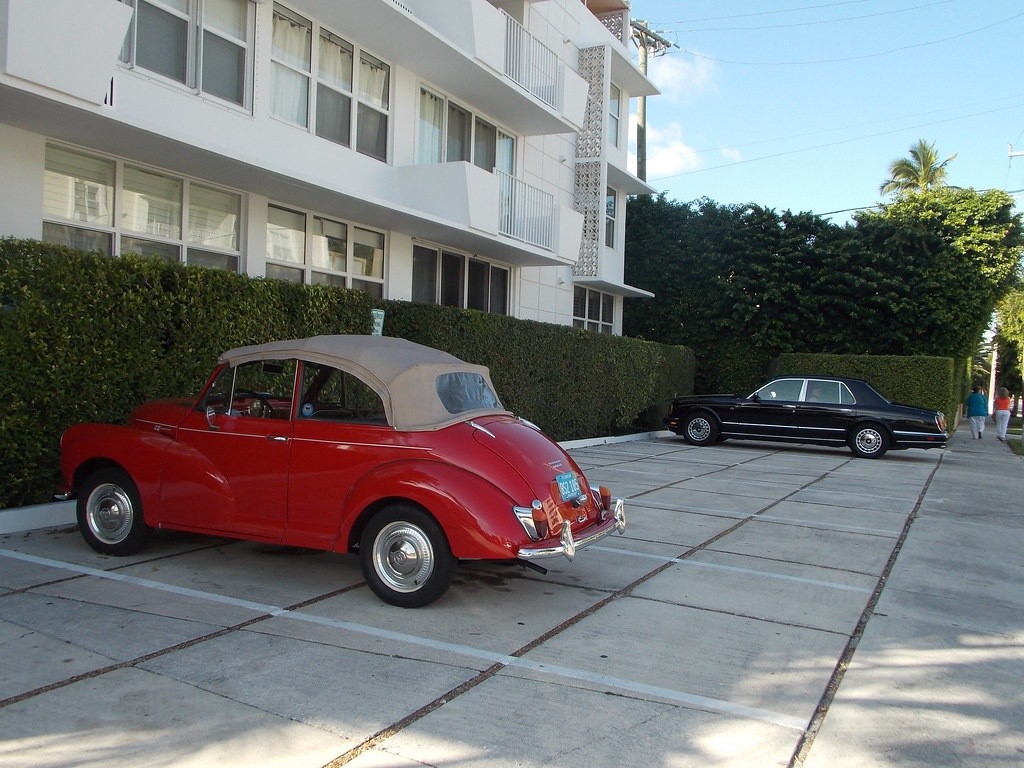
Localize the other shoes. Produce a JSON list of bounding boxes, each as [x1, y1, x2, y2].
[[978, 431, 982, 439], [998, 437, 1005, 443]]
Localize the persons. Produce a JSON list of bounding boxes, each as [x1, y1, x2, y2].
[[994, 387, 1011, 441], [966, 385, 988, 440]]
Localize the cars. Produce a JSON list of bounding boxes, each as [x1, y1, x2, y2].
[[663, 375, 951, 461], [58, 332, 627, 612]]
